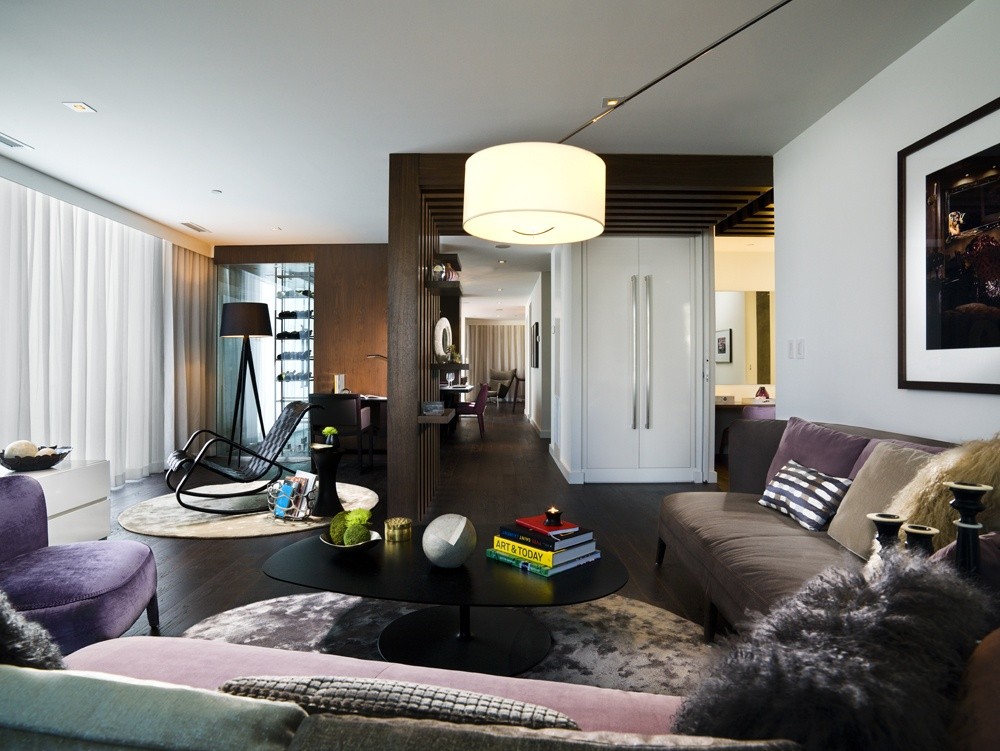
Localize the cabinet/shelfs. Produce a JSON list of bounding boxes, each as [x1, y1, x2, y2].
[[0, 459, 111, 546], [429, 254, 464, 296], [271, 263, 315, 459]]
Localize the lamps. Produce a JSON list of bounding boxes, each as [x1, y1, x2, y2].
[[218, 302, 273, 471], [463, 139, 607, 245]]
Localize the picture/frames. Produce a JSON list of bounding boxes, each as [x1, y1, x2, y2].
[[897, 94, 1000, 395], [529, 322, 538, 369], [715, 328, 732, 363]]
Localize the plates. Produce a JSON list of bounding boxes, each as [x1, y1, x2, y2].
[[319, 531, 382, 548], [0, 445, 73, 472]]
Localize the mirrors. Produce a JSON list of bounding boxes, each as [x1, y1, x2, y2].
[[714, 290, 771, 385]]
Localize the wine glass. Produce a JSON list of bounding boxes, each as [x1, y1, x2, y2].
[[446, 372, 455, 388]]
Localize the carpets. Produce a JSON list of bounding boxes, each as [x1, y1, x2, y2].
[[117, 476, 379, 539], [177, 590, 732, 698]]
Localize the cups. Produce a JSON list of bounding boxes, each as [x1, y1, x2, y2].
[[423, 401, 445, 416]]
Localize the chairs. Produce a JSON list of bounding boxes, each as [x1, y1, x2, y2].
[[0, 475, 159, 658], [479, 368, 517, 408], [447, 383, 488, 439], [164, 400, 316, 514]]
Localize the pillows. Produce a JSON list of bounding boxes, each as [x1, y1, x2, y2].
[[758, 416, 950, 562], [221, 674, 579, 730]]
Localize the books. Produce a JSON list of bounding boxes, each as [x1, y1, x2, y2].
[[273, 470, 318, 521], [484, 513, 602, 579]]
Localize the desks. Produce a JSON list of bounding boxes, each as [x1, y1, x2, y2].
[[261, 519, 629, 676], [440, 383, 474, 393]]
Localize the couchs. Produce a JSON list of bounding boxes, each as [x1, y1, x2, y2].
[[656, 413, 1000, 634], [0, 632, 797, 751]]
